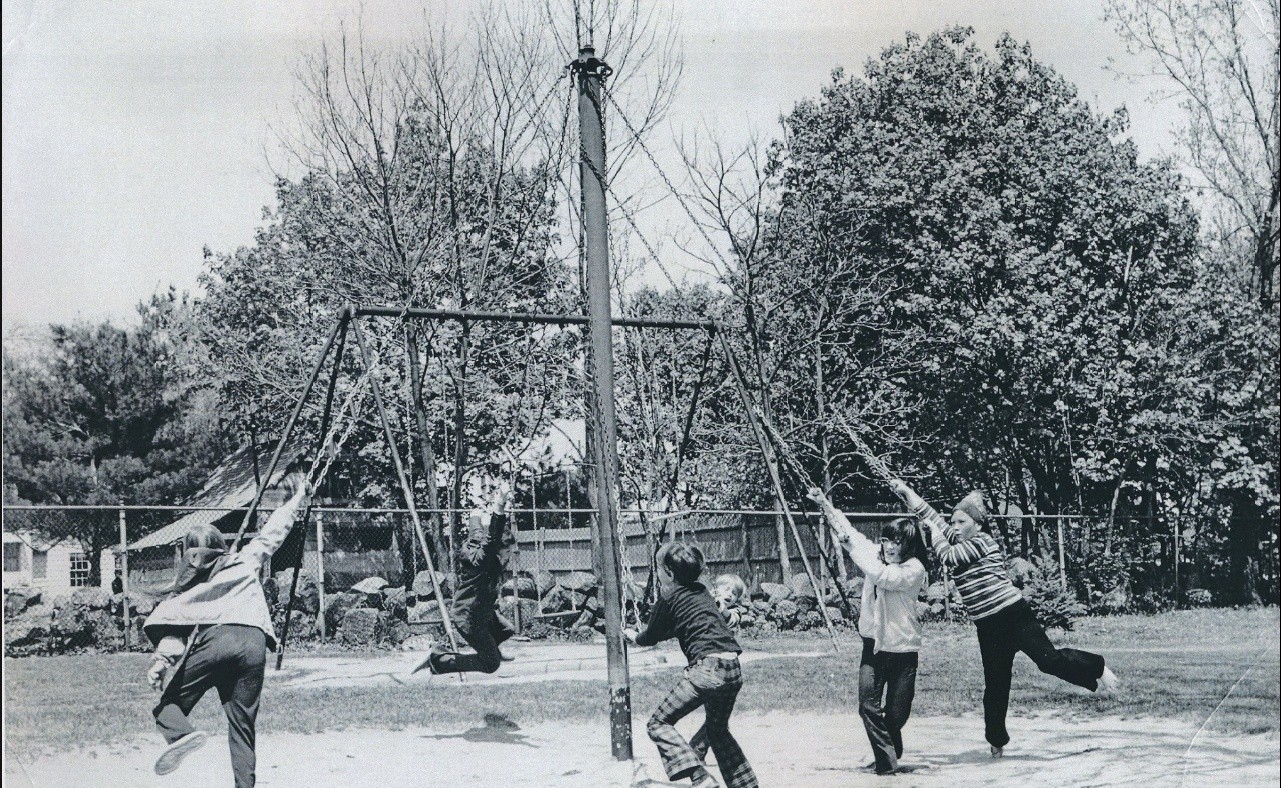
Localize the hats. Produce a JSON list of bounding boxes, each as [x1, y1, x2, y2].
[[952, 489, 987, 525]]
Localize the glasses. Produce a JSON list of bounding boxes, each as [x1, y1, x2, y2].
[[879, 537, 904, 549]]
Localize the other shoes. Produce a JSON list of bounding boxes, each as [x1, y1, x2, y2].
[[155, 729, 207, 776], [411, 658, 430, 675], [691, 774, 720, 788], [496, 648, 515, 662]]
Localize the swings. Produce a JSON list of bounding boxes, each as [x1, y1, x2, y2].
[[402, 308, 459, 626], [522, 310, 582, 620]]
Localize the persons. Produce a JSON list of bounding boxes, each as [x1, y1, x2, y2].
[[412, 488, 518, 676], [806, 487, 926, 775], [623, 543, 762, 788], [142, 478, 310, 788], [892, 480, 1120, 758]]
